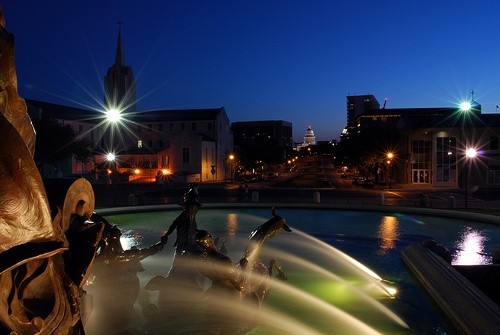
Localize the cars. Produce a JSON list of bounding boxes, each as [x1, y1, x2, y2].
[[364, 180, 374, 188], [341, 174, 347, 178]]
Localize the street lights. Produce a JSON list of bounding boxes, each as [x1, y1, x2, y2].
[[387, 153, 393, 188], [105, 152, 115, 185], [464, 144, 477, 210], [229, 155, 234, 183]]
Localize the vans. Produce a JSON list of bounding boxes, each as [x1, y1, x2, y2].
[[355, 177, 366, 184]]
